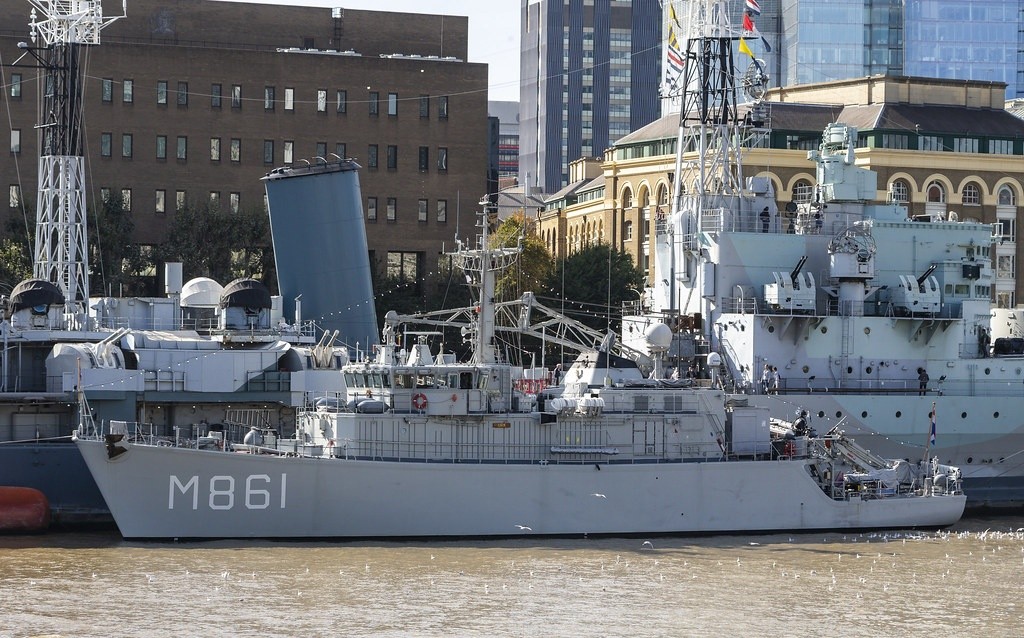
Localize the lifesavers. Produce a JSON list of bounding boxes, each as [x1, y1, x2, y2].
[[413, 393, 427, 410], [783, 441, 797, 456]]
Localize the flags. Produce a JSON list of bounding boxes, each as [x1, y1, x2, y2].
[[739, 38, 764, 77], [668, 50, 685, 90], [931, 406, 937, 445], [746, 0, 762, 16], [742, 12, 772, 52]]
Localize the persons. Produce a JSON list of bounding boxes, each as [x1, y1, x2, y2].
[[814, 206, 823, 235], [762, 363, 781, 395], [979, 329, 991, 358], [552, 363, 561, 385], [918, 370, 929, 396], [792, 410, 810, 437], [760, 206, 771, 233]]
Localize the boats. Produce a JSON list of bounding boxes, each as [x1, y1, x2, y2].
[[71, 195, 969, 541]]
